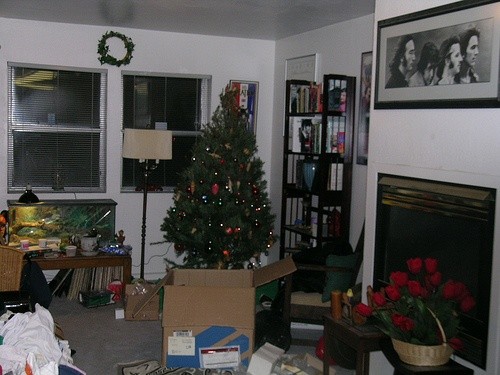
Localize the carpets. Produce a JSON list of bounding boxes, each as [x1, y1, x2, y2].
[[118, 350, 237, 375]]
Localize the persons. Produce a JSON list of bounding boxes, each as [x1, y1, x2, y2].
[[435, 37, 463, 85], [407, 42, 437, 87], [454, 29, 481, 84], [385, 35, 416, 88]]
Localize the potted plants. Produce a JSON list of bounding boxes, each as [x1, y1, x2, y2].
[[67, 209, 103, 256]]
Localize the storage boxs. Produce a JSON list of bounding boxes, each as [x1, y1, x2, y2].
[[126, 277, 169, 322], [80, 290, 116, 309], [161, 257, 296, 367], [5, 198, 119, 248], [246, 342, 285, 375]]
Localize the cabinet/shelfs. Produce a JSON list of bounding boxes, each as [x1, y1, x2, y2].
[[280, 74, 353, 261]]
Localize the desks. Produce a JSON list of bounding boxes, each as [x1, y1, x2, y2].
[[321, 313, 386, 375], [381, 345, 474, 375], [24, 253, 132, 300]]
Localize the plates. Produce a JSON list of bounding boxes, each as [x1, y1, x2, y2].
[[81, 252, 99, 256]]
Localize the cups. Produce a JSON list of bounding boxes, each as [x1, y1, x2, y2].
[[20, 240, 30, 250], [38, 239, 47, 248], [65, 246, 76, 257]]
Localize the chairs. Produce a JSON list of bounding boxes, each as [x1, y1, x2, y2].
[[278, 228, 368, 344]]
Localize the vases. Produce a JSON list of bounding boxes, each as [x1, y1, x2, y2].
[[391, 340, 454, 366]]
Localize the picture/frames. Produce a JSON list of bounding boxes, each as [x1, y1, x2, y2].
[[230, 79, 259, 142], [285, 54, 319, 81], [373, 0, 500, 108], [356, 52, 373, 166]]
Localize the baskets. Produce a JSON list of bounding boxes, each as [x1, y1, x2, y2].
[[392, 306, 454, 366]]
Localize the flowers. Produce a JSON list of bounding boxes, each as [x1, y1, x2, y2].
[[357, 257, 470, 349]]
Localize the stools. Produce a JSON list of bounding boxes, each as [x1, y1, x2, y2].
[[0, 289, 37, 315]]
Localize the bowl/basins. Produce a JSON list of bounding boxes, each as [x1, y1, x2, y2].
[[81, 236, 100, 252]]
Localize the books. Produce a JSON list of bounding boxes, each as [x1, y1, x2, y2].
[[289, 83, 346, 154]]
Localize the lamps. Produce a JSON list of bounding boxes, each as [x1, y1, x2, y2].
[[53, 170, 76, 199], [124, 128, 176, 283], [16, 182, 39, 203]]
